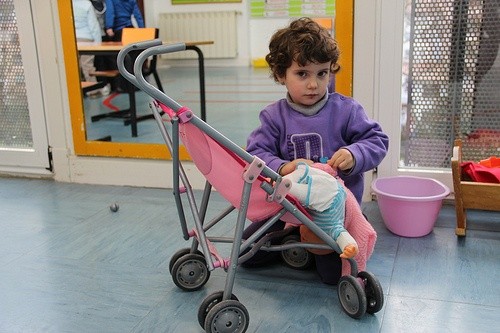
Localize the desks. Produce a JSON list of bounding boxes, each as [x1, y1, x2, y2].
[[81, 41, 145, 137]]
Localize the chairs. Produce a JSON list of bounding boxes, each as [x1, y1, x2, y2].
[[79, 82, 111, 142], [91, 28, 166, 128]]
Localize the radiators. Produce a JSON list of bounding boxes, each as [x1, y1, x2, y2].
[[154, 11, 241, 58]]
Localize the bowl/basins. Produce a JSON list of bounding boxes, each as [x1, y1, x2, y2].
[[370, 175, 451, 238]]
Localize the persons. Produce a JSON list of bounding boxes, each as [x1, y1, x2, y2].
[[279, 160, 360, 258], [232, 16, 389, 286], [71, 0, 146, 99]]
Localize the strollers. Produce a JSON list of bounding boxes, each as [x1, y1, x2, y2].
[[117, 38, 384, 333]]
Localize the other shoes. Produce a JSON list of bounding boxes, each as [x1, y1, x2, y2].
[[116, 86, 130, 93]]
